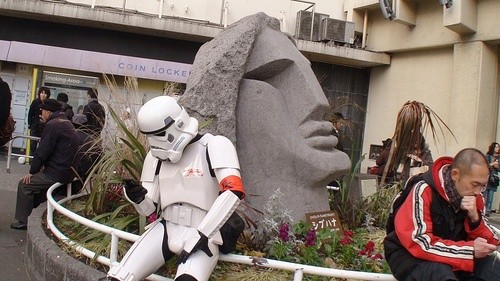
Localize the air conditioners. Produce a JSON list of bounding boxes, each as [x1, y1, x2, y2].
[[294, 10, 330, 42], [321, 18, 356, 44]]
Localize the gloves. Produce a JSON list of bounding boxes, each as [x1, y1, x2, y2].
[[175, 228, 213, 265], [124, 179, 148, 204]]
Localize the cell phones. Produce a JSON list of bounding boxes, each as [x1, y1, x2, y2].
[[124, 179, 138, 189]]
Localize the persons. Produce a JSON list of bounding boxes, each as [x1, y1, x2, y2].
[[10, 98, 80, 230], [389, 132, 435, 180], [77, 105, 85, 114], [105, 95, 246, 281], [0, 76, 12, 127], [383, 148, 500, 281], [183, 12, 352, 223], [329, 110, 345, 196], [484, 142, 500, 218], [83, 88, 106, 174], [0, 111, 15, 146], [66, 112, 95, 196], [28, 87, 51, 176], [56, 93, 74, 121]]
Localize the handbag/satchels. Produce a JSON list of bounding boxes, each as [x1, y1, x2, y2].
[[490, 175, 500, 186], [219, 212, 245, 255]]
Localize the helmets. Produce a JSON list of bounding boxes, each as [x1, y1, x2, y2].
[[137, 95, 199, 164]]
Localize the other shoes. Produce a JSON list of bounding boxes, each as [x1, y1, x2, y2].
[[11, 222, 27, 230]]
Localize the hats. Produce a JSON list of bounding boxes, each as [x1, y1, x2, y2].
[[72, 113, 88, 125], [57, 93, 68, 102], [37, 99, 62, 112]]
